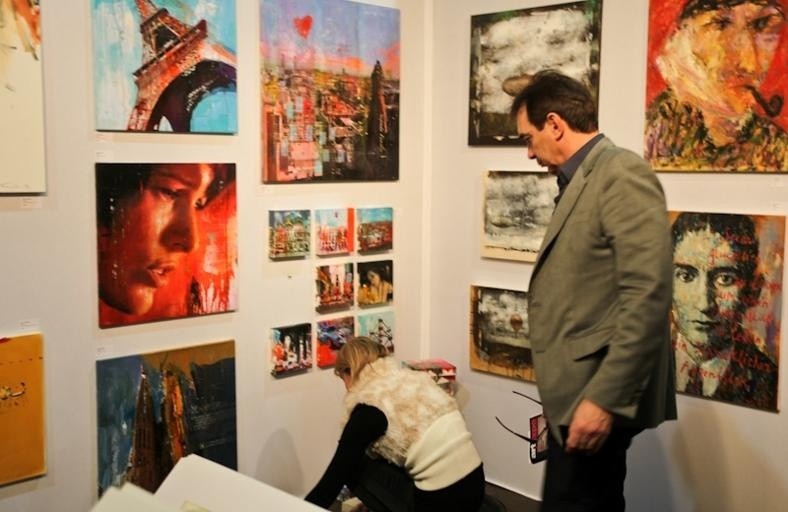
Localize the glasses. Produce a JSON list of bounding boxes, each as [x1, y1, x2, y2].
[[495, 391, 548, 444]]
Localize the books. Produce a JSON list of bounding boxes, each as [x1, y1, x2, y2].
[[529, 414, 552, 464]]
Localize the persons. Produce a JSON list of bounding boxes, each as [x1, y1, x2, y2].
[[284, 334, 297, 368], [303, 336, 485, 512], [662, 213, 778, 410], [98, 165, 233, 317], [357, 267, 393, 305], [511, 69, 674, 512], [647, 0, 787, 173]]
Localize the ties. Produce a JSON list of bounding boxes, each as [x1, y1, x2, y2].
[[684, 367, 702, 396]]
